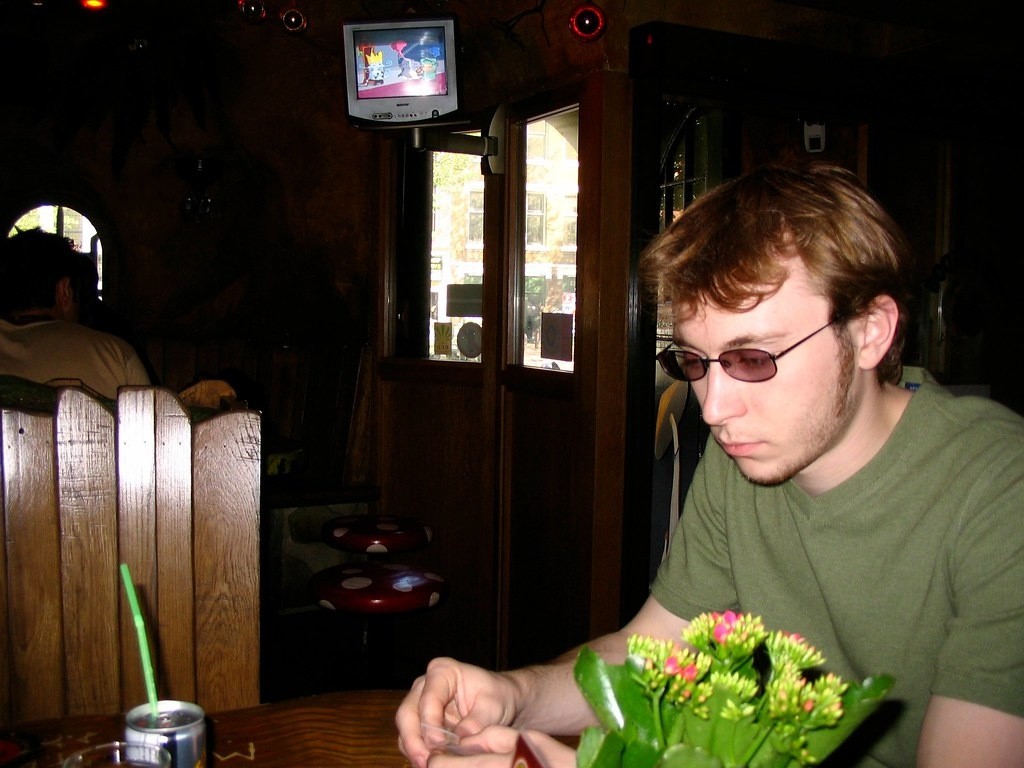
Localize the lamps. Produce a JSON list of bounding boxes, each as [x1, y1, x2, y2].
[[237, 0, 308, 36], [568, 0, 607, 41]]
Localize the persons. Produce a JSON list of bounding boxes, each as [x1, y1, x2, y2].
[[0, 229, 151, 400], [394, 163, 1024, 768]]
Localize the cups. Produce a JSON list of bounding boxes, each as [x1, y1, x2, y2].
[[62, 741, 171, 768]]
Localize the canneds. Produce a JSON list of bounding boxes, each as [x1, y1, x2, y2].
[[124, 700, 207, 768]]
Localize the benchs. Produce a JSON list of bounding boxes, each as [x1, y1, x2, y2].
[[0, 385, 261, 727]]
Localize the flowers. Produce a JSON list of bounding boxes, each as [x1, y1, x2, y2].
[[574, 610, 895, 768]]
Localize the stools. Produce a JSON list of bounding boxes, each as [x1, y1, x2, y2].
[[312, 515, 441, 663]]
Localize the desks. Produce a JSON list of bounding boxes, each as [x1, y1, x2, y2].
[[0, 689, 415, 768]]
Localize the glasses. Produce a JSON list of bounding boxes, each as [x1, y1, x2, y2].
[[656, 319, 838, 383]]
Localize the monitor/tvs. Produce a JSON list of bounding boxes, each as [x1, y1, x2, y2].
[[342, 18, 458, 126]]
